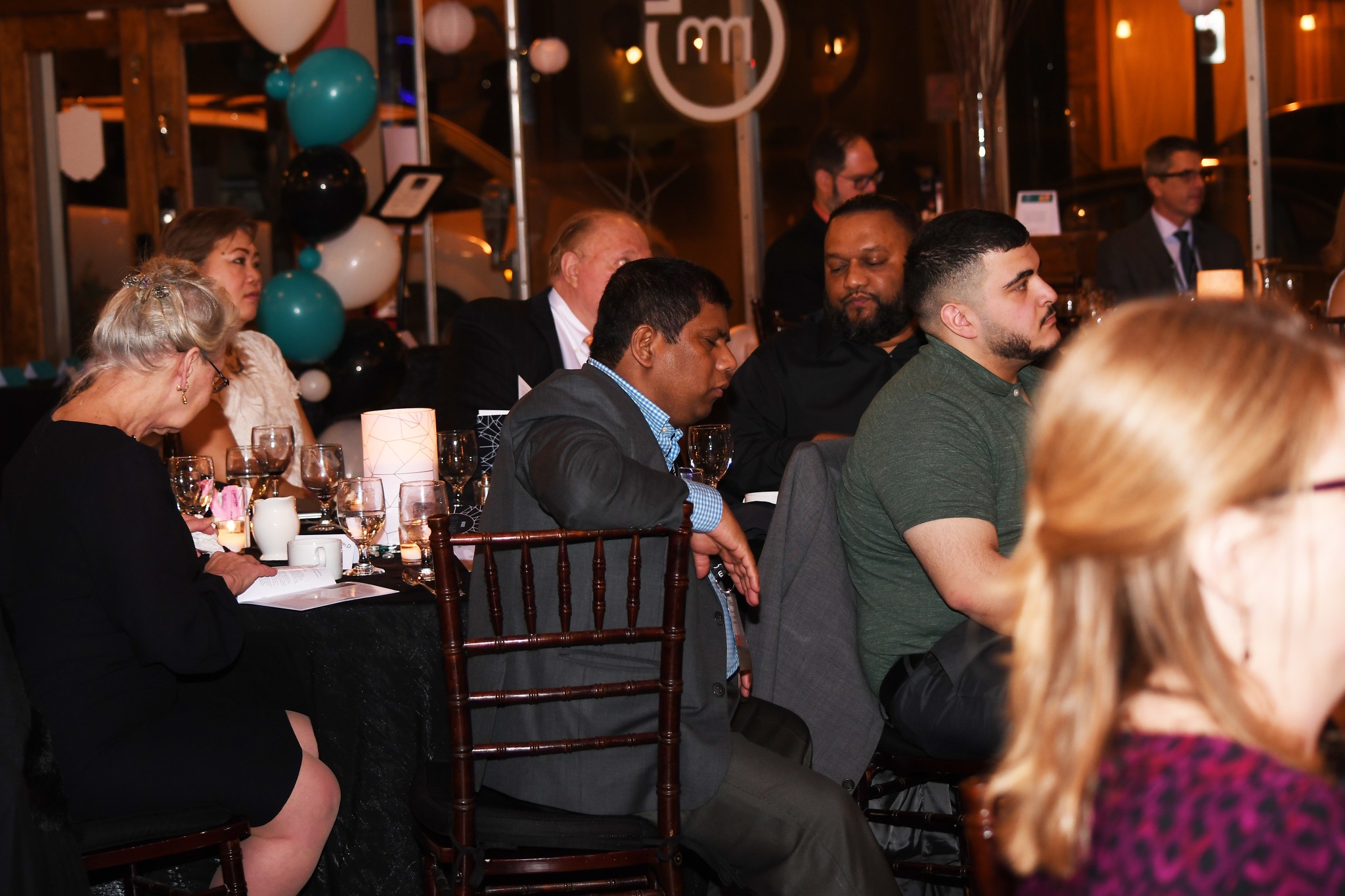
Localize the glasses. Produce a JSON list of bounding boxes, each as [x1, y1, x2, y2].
[[176, 347, 230, 394], [1161, 169, 1217, 184], [829, 168, 883, 190]]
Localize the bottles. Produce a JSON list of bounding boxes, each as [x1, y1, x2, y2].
[[480, 473, 491, 506], [1255, 258, 1291, 308]]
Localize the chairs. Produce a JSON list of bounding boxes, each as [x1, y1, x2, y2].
[[738, 437, 1030, 896], [417, 500, 697, 896]]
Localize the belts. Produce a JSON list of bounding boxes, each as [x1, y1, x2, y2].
[[880, 654, 924, 715]]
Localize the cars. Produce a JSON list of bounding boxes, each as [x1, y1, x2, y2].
[[58, 87, 680, 304]]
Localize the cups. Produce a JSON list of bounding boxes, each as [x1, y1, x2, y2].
[[679, 467, 704, 484], [169, 457, 215, 517], [251, 496, 301, 560], [216, 520, 245, 556], [287, 538, 343, 580], [398, 520, 429, 566], [745, 491, 779, 504]]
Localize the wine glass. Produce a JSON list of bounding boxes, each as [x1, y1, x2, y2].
[[687, 425, 734, 489], [300, 444, 344, 532], [225, 445, 270, 533], [337, 477, 386, 576], [399, 480, 450, 581], [437, 430, 478, 505], [251, 424, 295, 498]]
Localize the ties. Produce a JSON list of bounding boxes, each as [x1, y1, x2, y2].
[[585, 334, 593, 350], [1173, 232, 1201, 288]]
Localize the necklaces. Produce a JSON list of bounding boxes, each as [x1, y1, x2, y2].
[[1140, 682, 1198, 697]]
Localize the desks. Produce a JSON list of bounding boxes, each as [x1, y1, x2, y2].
[[194, 510, 486, 896]]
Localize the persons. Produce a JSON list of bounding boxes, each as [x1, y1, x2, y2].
[[1, 254, 341, 896], [464, 257, 900, 896], [709, 193, 924, 499], [156, 207, 321, 501], [765, 131, 883, 322], [1094, 135, 1243, 300], [966, 293, 1345, 896], [838, 208, 1062, 763], [459, 209, 651, 463]]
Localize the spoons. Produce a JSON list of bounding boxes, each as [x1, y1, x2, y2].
[[402, 568, 437, 595]]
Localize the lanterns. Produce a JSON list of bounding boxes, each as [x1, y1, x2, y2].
[[425, 3, 476, 55], [528, 38, 568, 73]]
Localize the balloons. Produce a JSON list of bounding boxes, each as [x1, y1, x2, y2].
[[288, 46, 375, 146], [228, 0, 335, 61], [265, 70, 293, 100], [254, 271, 343, 362], [299, 370, 331, 402], [298, 246, 320, 270], [282, 146, 368, 242], [313, 217, 401, 311]]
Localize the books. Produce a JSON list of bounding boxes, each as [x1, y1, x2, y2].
[[236, 565, 400, 611]]
